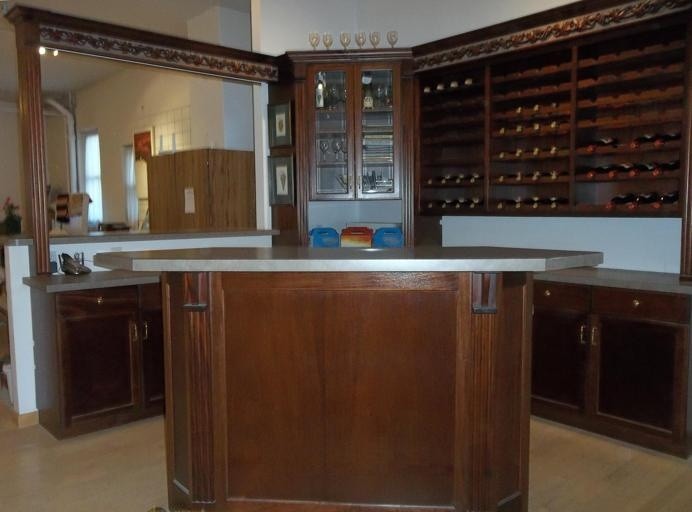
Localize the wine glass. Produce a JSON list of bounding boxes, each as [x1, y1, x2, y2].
[[332, 140, 342, 161], [308, 31, 398, 50], [319, 140, 328, 162]]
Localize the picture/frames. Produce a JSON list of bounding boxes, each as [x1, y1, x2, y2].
[[267, 98, 294, 148], [267, 153, 294, 207], [132, 127, 155, 162]]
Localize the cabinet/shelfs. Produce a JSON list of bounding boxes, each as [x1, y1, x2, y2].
[[487, 63, 572, 211], [30, 282, 165, 440], [575, 39, 683, 211], [147, 149, 256, 234], [419, 81, 488, 212], [307, 62, 401, 200], [531, 281, 691, 460]]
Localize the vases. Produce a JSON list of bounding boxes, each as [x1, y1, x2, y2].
[[3, 214, 19, 234]]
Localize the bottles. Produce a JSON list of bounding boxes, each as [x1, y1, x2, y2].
[[605, 191, 679, 212], [587, 136, 619, 153], [629, 130, 681, 149], [587, 160, 676, 178]]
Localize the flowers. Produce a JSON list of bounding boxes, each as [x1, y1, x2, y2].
[[0, 194, 19, 217]]
